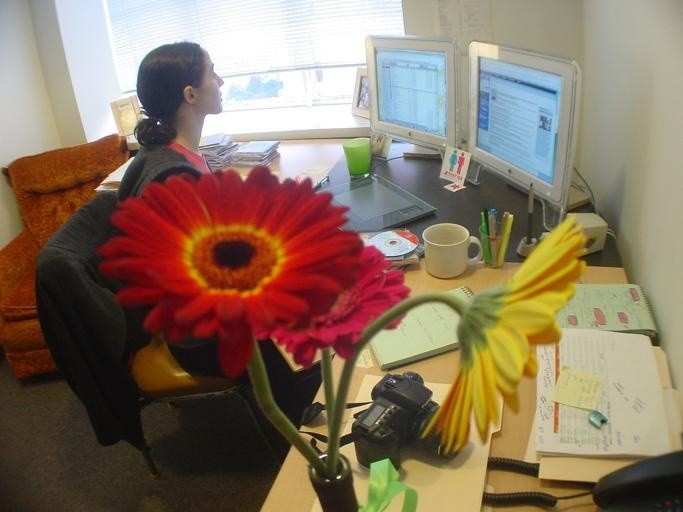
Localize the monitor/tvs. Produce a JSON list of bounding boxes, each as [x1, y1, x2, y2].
[[366, 35, 462, 161], [467, 40, 592, 211]]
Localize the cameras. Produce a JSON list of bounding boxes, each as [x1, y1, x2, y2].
[[352, 371, 460, 471]]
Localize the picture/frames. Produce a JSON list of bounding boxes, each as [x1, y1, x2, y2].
[[108, 95, 143, 137], [350, 67, 369, 119]]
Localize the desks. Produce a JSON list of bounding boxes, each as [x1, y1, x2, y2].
[[214, 137, 681, 510]]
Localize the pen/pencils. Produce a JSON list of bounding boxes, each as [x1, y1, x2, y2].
[[480, 208, 514, 267]]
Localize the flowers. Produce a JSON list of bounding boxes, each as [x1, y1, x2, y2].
[[93, 160, 590, 477]]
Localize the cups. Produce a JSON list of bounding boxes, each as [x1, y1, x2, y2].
[[342, 138, 371, 178], [421, 222, 483, 279]]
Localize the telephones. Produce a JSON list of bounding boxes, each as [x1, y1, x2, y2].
[[593, 451, 683, 512]]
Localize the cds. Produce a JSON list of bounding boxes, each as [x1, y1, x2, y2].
[[368, 229, 419, 256]]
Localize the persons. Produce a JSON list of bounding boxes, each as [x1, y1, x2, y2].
[[116, 41, 224, 200]]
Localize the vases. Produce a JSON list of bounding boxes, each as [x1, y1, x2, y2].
[[307, 453, 365, 510]]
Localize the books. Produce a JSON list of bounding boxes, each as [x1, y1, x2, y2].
[[199, 134, 281, 169], [369, 285, 476, 369]]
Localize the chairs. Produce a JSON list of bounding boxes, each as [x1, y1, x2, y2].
[[34, 186, 277, 473], [1, 132, 132, 384]]
